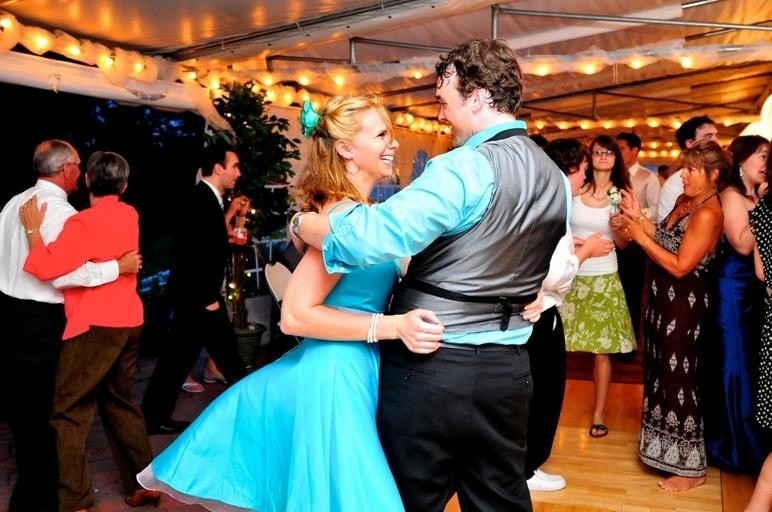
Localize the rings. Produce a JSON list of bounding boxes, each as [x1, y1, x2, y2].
[[139, 265, 143, 269]]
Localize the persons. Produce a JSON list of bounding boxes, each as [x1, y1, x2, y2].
[[527, 139, 615, 492], [182, 165, 252, 393], [0, 137, 143, 512], [744, 138, 772, 512], [618, 137, 723, 491], [570, 115, 719, 437], [137, 145, 245, 437], [135, 93, 544, 512], [704, 134, 772, 477], [287, 35, 570, 512], [18, 149, 161, 512]]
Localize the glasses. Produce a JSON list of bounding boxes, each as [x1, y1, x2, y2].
[[591, 150, 614, 155]]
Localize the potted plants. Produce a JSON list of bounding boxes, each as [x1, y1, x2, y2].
[[199, 81, 304, 366]]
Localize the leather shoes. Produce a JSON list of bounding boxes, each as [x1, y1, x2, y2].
[[148, 421, 190, 436]]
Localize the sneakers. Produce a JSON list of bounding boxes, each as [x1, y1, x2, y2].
[[526, 469, 566, 492]]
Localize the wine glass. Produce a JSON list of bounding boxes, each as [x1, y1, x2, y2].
[[234, 200, 250, 239]]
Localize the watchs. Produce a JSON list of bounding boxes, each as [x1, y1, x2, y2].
[[292, 211, 307, 240]]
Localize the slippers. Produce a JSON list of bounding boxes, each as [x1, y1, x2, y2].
[[181, 383, 204, 393], [590, 425, 608, 437]]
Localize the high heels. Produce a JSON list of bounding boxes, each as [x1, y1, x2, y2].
[[125, 490, 160, 507]]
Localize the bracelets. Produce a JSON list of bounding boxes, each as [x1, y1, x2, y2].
[[366, 312, 383, 343], [25, 228, 39, 238]]
